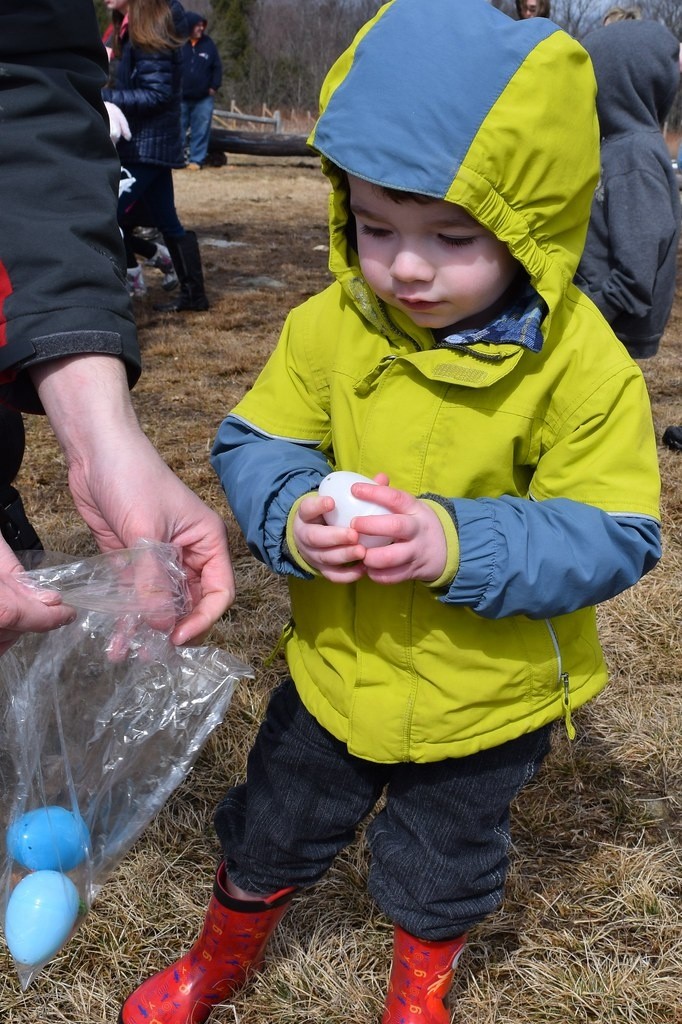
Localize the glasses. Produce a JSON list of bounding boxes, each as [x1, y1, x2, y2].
[[521, 4, 540, 12]]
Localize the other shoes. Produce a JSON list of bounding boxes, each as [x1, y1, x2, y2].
[[662, 426, 682, 452], [188, 162, 201, 171], [0, 496, 45, 572]]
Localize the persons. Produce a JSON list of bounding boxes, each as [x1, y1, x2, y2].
[[0, 1, 235, 648], [112, 1, 682, 1024]]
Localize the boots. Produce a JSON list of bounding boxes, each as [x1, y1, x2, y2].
[[125, 263, 145, 302], [119, 860, 299, 1024], [144, 240, 179, 292], [383, 923, 468, 1024], [154, 231, 210, 313]]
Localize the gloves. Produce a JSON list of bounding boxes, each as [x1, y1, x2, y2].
[[103, 100, 132, 150]]
